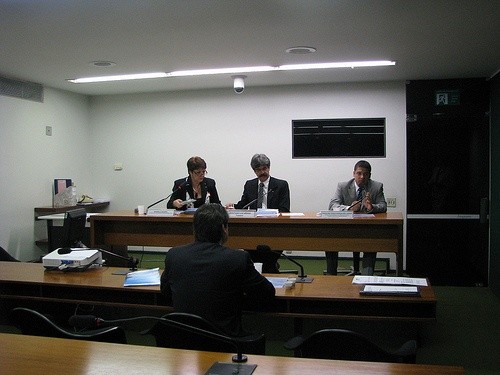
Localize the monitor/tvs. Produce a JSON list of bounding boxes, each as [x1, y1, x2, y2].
[[61, 208, 87, 249]]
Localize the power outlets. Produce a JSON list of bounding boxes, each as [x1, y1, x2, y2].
[[386, 198, 396, 207]]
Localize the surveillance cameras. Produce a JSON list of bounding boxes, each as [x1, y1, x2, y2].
[[233, 78, 245, 94]]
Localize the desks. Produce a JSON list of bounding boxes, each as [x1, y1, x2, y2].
[[0, 261, 440, 320], [90, 209, 404, 275], [0, 334, 463, 375]]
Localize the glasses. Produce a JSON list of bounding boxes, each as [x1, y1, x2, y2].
[[192, 169, 208, 176]]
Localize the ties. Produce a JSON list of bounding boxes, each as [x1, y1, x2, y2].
[[356, 187, 365, 207], [258, 182, 264, 208]]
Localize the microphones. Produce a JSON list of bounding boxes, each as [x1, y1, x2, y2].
[[69, 315, 243, 357], [256, 245, 303, 280], [243, 189, 273, 209], [58, 248, 136, 270], [144, 181, 188, 215], [347, 188, 367, 211]]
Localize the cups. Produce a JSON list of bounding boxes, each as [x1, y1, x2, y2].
[[253, 262, 262, 274], [138, 206, 144, 215]]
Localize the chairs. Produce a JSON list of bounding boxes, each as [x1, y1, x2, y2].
[[149, 318, 266, 352], [295, 328, 417, 363]]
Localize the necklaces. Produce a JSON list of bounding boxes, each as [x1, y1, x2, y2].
[[193, 185, 201, 197]]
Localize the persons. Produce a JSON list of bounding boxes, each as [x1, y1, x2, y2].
[[225, 153, 290, 273], [325, 161, 387, 275], [167, 157, 222, 212], [160, 202, 277, 336]]
